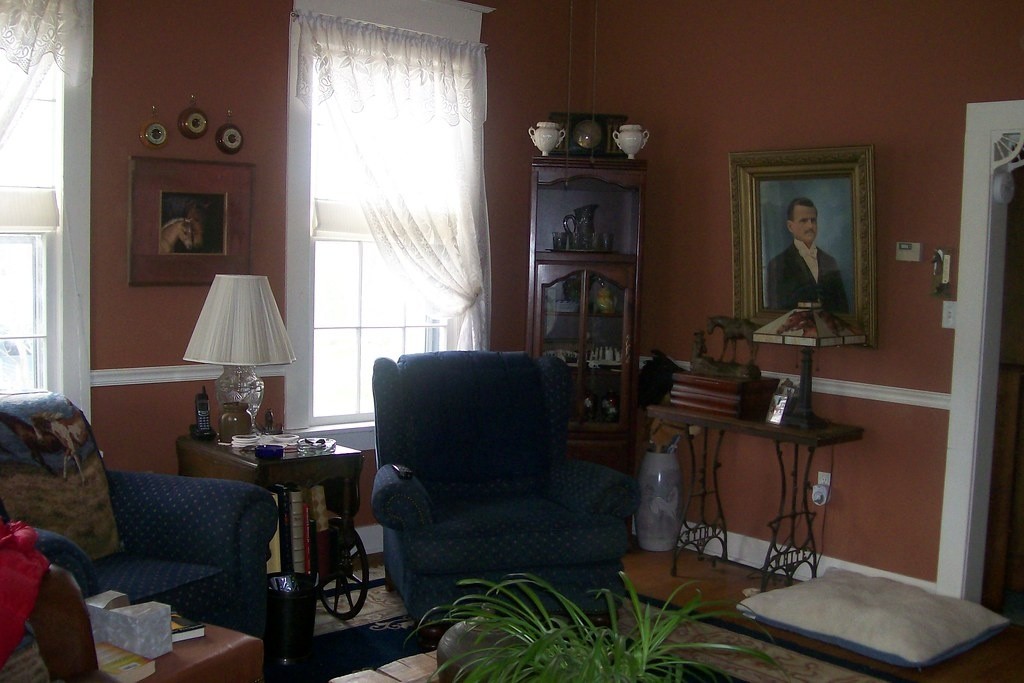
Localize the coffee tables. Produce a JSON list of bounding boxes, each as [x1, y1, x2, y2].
[[174, 428, 368, 621]]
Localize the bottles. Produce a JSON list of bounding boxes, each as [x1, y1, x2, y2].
[[218, 401, 251, 443], [633, 450, 683, 552], [265, 408, 273, 432]]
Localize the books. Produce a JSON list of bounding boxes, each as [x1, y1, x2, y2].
[[96, 642, 155, 683], [171, 612, 207, 644]]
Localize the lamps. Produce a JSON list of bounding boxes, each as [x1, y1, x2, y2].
[[182, 274, 300, 433], [751, 300, 868, 430]]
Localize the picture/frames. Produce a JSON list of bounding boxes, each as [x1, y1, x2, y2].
[[126, 155, 257, 286], [726, 143, 879, 351]]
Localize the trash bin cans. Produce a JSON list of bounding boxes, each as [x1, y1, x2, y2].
[[263, 571, 316, 665]]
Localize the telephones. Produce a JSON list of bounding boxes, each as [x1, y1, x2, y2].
[[189, 385, 217, 441]]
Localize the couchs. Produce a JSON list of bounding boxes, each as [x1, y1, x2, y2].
[[0, 391, 279, 642], [369, 351, 642, 650]]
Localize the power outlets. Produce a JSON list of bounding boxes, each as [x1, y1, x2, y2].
[[817, 471, 832, 487]]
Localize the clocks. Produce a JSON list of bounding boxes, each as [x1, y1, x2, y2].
[[564, 111, 608, 156]]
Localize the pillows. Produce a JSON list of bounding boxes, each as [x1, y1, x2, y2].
[[735, 567, 1011, 669]]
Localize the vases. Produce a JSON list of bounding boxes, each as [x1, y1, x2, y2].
[[632, 449, 683, 552]]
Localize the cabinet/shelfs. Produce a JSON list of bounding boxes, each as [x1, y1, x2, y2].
[[524, 156, 648, 550]]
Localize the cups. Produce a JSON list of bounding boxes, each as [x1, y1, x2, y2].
[[570, 232, 613, 250], [552, 232, 567, 249]]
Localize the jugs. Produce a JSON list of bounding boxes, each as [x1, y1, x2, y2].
[[562, 204, 599, 250]]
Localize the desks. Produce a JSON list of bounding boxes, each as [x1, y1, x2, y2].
[[646, 402, 864, 592], [138, 620, 264, 683]]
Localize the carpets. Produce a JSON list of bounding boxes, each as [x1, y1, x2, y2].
[[263, 564, 912, 683]]
